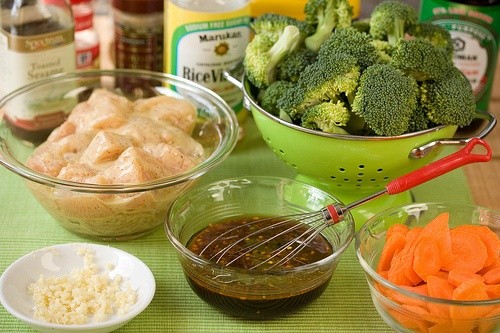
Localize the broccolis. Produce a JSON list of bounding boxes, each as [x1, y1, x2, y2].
[[242, 0, 492, 139]]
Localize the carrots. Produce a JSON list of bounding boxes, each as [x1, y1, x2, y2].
[[373, 211, 500, 333]]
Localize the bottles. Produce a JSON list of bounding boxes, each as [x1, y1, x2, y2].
[[416, 0, 500, 132], [162, 0, 252, 135], [0, 0, 79, 141], [42, 0, 100, 98], [112, 0, 165, 96]]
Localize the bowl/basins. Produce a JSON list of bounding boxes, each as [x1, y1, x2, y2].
[[0, 243, 156, 333], [165, 177, 356, 319], [355, 201, 500, 333], [0, 69, 238, 239]]
